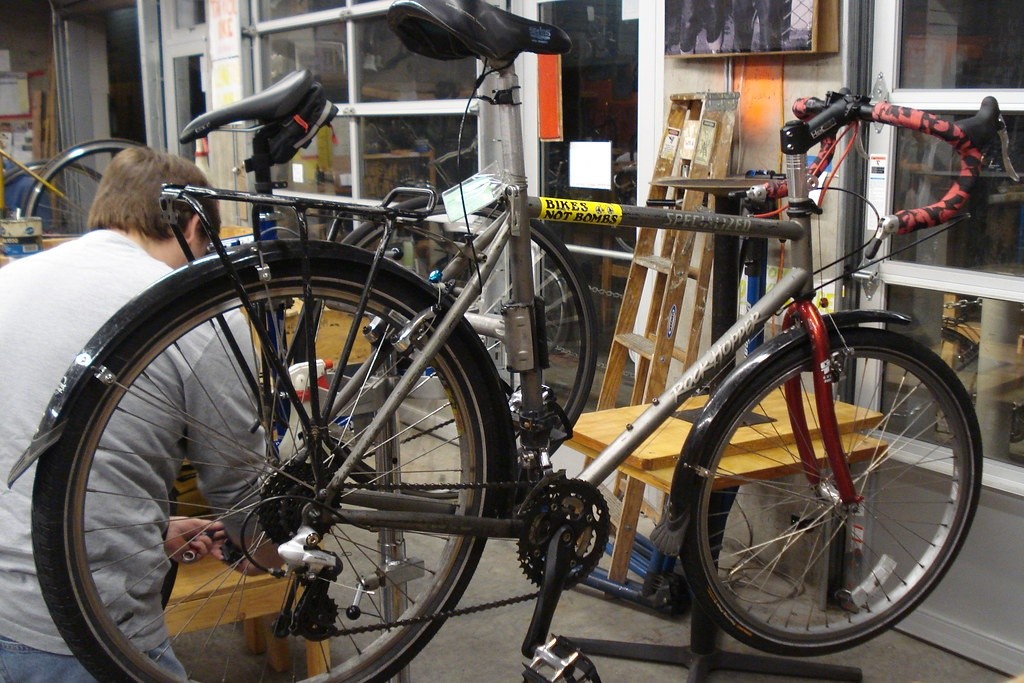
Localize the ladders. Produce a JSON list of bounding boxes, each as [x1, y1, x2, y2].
[[594, 89, 744, 601]]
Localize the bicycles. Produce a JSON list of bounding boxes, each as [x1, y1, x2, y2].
[[6, 0, 1020, 683]]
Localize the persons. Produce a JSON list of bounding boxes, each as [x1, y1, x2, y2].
[[0, 143, 298, 683], [667, 0, 806, 60]]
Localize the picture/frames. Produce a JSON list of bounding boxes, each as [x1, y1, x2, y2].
[[664, 1, 819, 60]]
